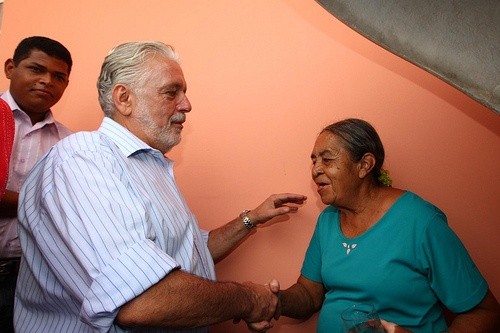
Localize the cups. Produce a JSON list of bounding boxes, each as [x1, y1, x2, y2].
[[341, 303, 385, 333]]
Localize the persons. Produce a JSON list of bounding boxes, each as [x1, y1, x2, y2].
[[0, 37, 76, 333], [251, 118, 500, 333], [15, 42, 307, 333]]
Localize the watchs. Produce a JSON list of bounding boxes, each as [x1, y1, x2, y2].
[[241, 210, 256, 229]]
